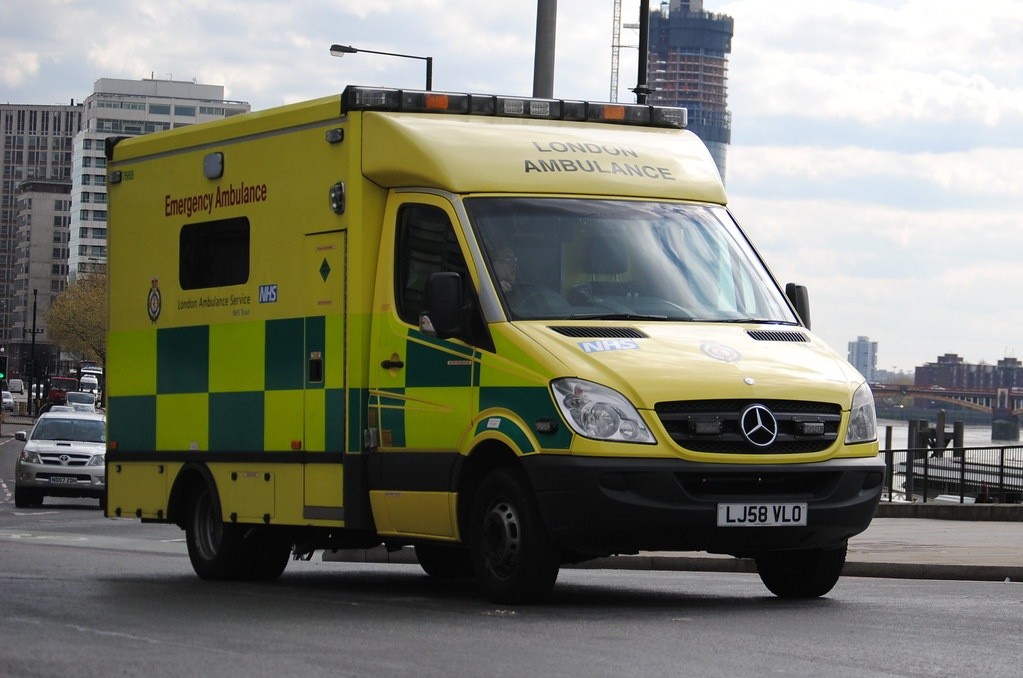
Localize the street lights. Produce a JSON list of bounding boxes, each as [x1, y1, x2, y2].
[[330, 42, 432, 93]]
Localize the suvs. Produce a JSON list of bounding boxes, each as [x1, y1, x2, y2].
[[14, 412, 106, 512]]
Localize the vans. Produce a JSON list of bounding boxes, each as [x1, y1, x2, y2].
[[1011, 386, 1023, 394]]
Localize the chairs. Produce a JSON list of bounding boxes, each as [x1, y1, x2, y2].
[[86, 427, 101, 442], [52, 422, 72, 439], [566, 235, 633, 306]]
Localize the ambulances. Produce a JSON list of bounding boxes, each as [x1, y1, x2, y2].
[[103, 84, 889, 601]]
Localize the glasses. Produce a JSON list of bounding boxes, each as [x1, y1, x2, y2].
[[492, 256, 520, 263]]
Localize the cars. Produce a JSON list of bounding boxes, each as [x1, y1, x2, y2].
[[0, 359, 105, 412], [930, 385, 946, 391], [868, 381, 885, 388]]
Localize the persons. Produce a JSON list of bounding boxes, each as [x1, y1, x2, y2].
[[471, 244, 518, 323]]
